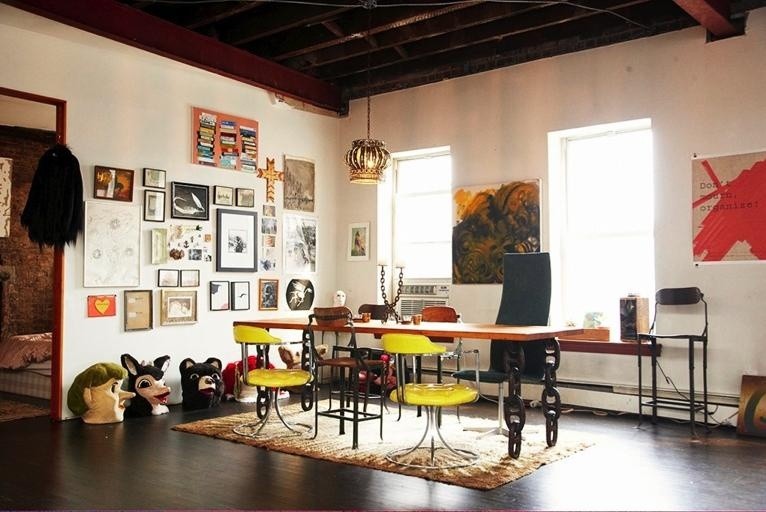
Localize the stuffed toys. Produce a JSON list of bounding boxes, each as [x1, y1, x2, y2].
[[278, 345, 330, 394], [120, 353, 172, 419], [67, 362, 136, 424]]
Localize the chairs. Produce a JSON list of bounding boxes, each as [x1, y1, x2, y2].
[[329, 302, 389, 415], [637, 287, 709, 439], [452, 252, 552, 438], [380, 333, 482, 469], [234, 325, 313, 437], [411, 306, 462, 427], [308, 307, 385, 449]]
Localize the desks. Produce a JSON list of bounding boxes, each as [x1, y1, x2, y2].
[[233, 317, 610, 460]]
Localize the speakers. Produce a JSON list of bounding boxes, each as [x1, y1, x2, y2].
[[619, 297, 650, 344]]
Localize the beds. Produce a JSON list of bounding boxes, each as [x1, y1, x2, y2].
[[0, 332, 51, 405]]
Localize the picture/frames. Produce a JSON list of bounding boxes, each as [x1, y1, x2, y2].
[[347, 222, 369, 260], [0, 158, 13, 239], [83, 165, 317, 331]]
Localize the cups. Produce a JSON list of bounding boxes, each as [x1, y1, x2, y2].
[[404, 314, 412, 322], [362, 313, 372, 323], [412, 314, 423, 325]]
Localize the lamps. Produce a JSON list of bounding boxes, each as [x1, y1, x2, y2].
[[345, 5, 391, 185]]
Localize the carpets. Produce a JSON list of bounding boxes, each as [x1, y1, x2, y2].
[[171, 397, 607, 492]]
